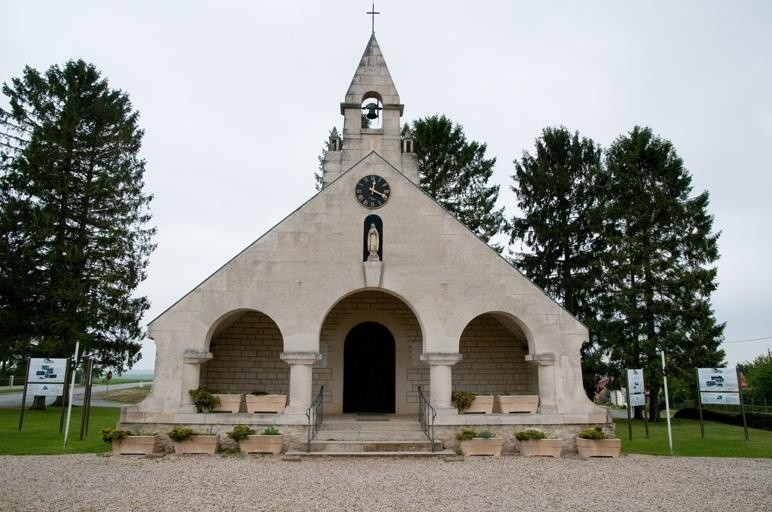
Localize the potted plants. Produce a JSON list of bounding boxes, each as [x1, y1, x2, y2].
[[453, 426, 502, 457], [576, 430, 621, 458], [512, 430, 562, 459], [226, 425, 285, 455], [451, 389, 494, 414], [246, 389, 288, 414], [188, 387, 243, 413], [102, 428, 161, 455], [498, 390, 539, 414], [167, 426, 220, 457]]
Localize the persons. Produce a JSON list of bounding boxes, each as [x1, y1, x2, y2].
[[367, 224, 380, 255]]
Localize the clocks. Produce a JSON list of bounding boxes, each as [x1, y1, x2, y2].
[[355, 175, 391, 209]]
